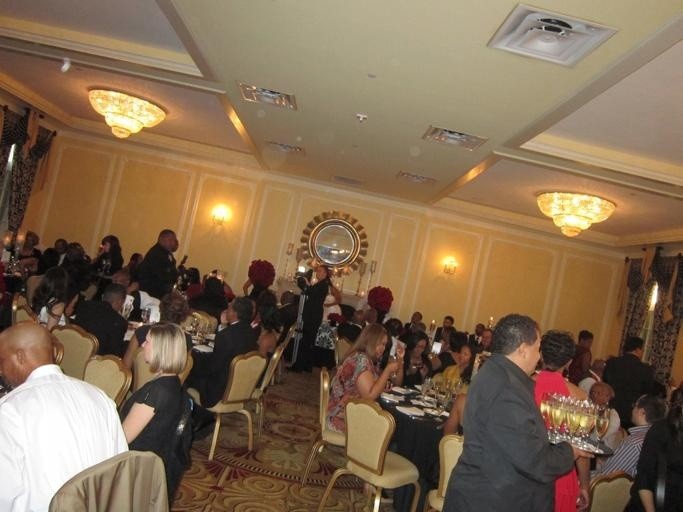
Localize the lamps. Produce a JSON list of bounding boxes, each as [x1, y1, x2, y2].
[[84, 84, 169, 140], [532, 189, 617, 240], [209, 203, 232, 227]]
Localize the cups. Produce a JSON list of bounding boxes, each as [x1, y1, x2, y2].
[[189, 312, 209, 344], [390, 356, 398, 378]]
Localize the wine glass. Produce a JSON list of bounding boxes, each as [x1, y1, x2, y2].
[[139, 306, 151, 326], [535, 390, 611, 453], [419, 374, 466, 415]]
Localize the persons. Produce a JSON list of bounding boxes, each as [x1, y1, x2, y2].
[[0, 229, 275, 512], [285, 264, 341, 375], [329, 310, 682, 511]]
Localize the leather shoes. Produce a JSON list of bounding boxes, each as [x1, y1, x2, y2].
[[283, 363, 314, 377], [191, 416, 220, 442]]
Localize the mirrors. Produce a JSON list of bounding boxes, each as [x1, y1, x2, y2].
[[307, 217, 362, 270]]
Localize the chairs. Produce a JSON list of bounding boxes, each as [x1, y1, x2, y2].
[[423, 434, 466, 511], [301, 365, 346, 486], [1, 275, 300, 511], [332, 333, 354, 370], [316, 399, 422, 512], [587, 469, 635, 512]]
